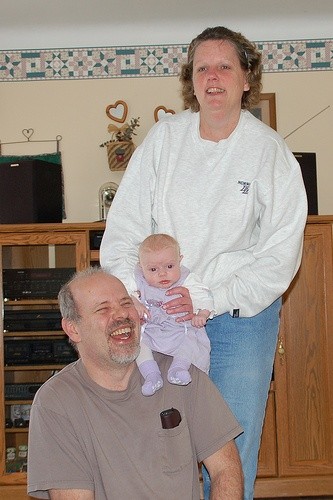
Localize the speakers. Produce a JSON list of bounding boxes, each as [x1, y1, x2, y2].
[[292, 152, 318, 215]]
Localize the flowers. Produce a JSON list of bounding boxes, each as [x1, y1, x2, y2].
[[99, 116, 140, 148]]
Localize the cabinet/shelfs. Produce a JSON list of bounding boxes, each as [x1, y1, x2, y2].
[[0, 214, 333, 500]]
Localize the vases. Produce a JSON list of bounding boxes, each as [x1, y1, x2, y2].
[[107, 142, 135, 171]]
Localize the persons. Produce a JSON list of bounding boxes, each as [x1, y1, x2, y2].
[[99, 26, 309, 500], [27, 268, 248, 500], [134, 233, 214, 396]]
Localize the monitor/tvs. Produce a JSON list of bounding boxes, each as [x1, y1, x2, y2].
[[0, 155, 62, 224]]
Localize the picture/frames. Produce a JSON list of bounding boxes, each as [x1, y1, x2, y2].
[[246, 92, 277, 133]]
